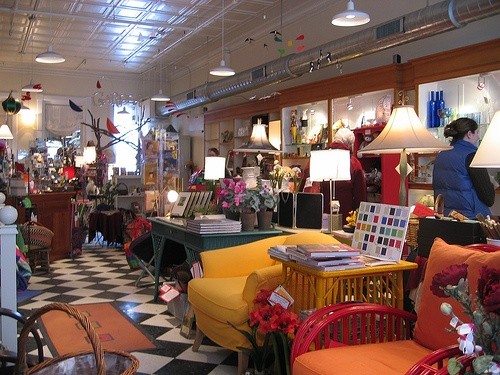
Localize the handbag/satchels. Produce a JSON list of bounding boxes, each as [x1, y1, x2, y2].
[[21, 221, 53, 249]]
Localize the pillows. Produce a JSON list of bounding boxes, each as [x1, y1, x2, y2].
[[412, 237, 500, 350]]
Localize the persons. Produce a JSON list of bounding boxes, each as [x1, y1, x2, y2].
[[298, 128, 368, 226], [432, 117, 495, 221], [206, 148, 230, 178]]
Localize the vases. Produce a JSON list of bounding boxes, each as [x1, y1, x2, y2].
[[226, 210, 239, 221]]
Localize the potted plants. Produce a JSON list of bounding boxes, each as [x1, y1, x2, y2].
[[241, 184, 282, 231]]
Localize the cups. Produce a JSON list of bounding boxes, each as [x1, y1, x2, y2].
[[121, 168, 126, 175], [113, 167, 119, 176]]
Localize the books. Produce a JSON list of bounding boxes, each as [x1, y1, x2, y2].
[[267, 243, 367, 272], [172, 190, 242, 234]]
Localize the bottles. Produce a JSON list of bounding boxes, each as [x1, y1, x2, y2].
[[429, 90, 445, 128]]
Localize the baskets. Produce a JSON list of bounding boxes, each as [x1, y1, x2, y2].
[[405, 194, 444, 246], [14, 302, 139, 375]]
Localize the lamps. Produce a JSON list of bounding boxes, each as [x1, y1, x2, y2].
[[18, 32, 43, 111], [204, 156, 226, 189], [151, 48, 171, 101], [0, 125, 14, 140], [232, 118, 281, 171], [331, 0, 370, 27], [310, 150, 351, 238], [209, 0, 235, 76], [35, 0, 66, 63], [359, 106, 454, 206]]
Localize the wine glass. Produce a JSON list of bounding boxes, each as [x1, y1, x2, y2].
[[437, 107, 452, 127]]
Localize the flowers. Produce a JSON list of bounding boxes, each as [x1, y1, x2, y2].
[[219, 176, 246, 210], [267, 159, 299, 195], [227, 289, 302, 375], [429, 265, 500, 375]]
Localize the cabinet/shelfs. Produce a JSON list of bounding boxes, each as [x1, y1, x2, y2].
[[3, 192, 73, 261], [153, 131, 190, 191], [203, 36, 500, 207], [112, 176, 145, 213]]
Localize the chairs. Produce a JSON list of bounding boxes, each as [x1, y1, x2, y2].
[[129, 233, 183, 286], [186, 227, 500, 375]]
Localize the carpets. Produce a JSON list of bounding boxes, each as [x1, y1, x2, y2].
[[32, 301, 162, 360]]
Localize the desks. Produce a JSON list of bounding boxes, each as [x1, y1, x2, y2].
[[147, 216, 284, 302], [87, 212, 124, 246]]
